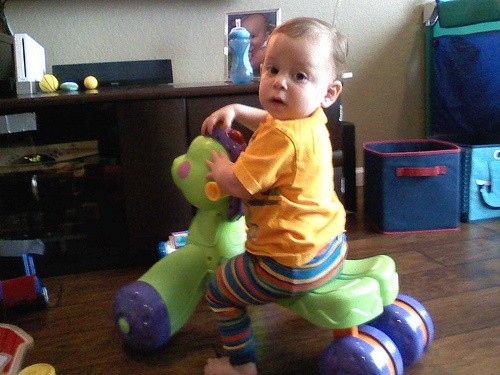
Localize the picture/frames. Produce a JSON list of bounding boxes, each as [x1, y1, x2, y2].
[[222, 7, 281, 82]]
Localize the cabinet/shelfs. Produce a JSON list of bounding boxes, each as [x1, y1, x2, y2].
[[0, 82, 358, 280]]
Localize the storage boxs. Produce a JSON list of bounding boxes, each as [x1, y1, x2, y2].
[[362, 137, 500, 235]]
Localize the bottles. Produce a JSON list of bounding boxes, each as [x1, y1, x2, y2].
[[228, 18, 253, 85]]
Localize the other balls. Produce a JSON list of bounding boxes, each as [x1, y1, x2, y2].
[[84, 76, 98, 89]]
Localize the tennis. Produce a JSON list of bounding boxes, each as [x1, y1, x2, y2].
[[39, 74, 59, 93]]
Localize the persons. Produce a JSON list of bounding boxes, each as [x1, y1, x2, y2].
[[241, 14, 271, 67], [201, 17, 349, 375]]
